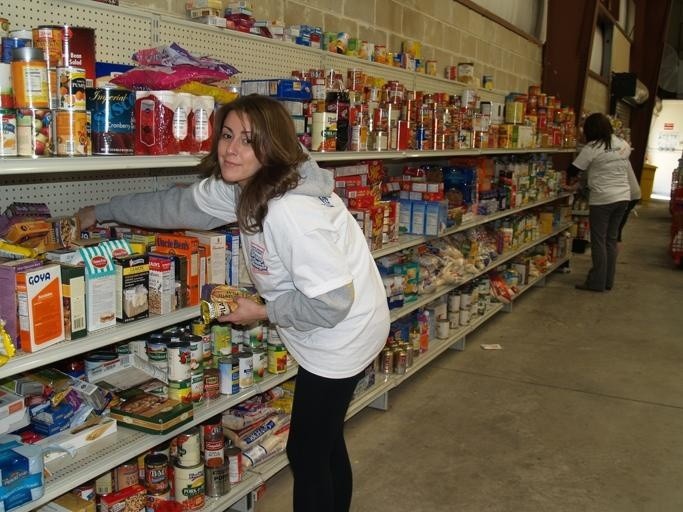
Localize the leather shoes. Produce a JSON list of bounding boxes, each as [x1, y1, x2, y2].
[[574, 283, 605, 293]]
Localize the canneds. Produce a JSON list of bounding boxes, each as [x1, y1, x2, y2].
[[307, 67, 491, 151], [146, 321, 289, 406], [0, 25, 88, 160], [94, 415, 243, 510], [529, 86, 578, 146], [377, 339, 415, 374]]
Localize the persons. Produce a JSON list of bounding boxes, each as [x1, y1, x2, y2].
[[565, 112, 632, 293], [616, 159, 642, 242], [72, 92, 392, 512]]
